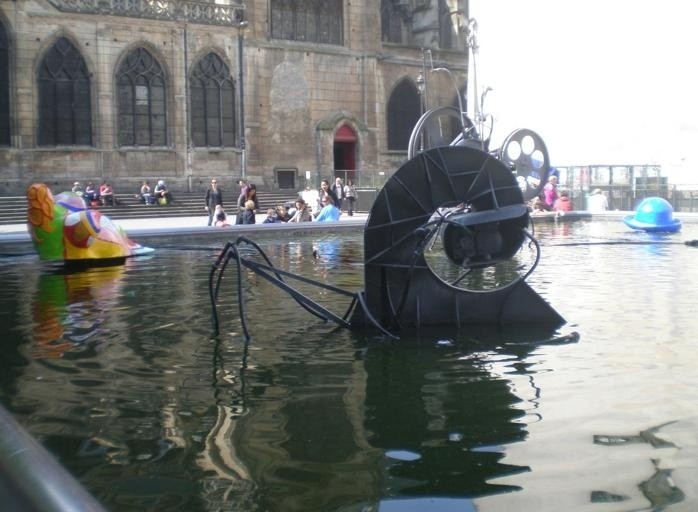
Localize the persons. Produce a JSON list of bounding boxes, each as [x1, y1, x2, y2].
[[525, 174, 573, 212], [136, 180, 154, 208], [154, 180, 174, 205], [72, 180, 118, 209], [587, 189, 608, 213], [205, 177, 357, 229]]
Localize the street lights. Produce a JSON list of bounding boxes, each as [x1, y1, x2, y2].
[[416, 73, 430, 155], [235, 22, 252, 181], [176, 20, 193, 190]]
